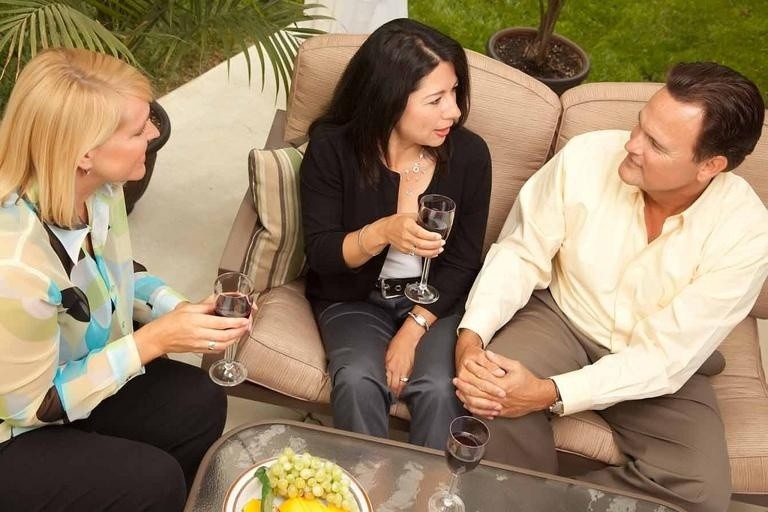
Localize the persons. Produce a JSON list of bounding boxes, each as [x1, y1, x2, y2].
[[0, 46, 258, 512], [453, 62, 767, 512], [302, 20, 493, 454]]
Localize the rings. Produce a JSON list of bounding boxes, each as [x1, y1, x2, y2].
[[400, 378, 409, 382], [410, 245, 416, 257], [208, 342, 215, 350]]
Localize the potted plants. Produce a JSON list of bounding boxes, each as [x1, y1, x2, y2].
[[1, 0, 340, 220]]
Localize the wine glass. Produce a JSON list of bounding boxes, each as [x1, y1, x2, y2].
[[209, 273, 255, 388], [429, 415, 491, 512], [404, 194, 455, 305]]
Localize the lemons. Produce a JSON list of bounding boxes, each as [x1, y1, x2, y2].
[[278, 497, 328, 512], [243, 500, 261, 512]]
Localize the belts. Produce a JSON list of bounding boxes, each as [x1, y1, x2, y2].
[[374, 277, 422, 299]]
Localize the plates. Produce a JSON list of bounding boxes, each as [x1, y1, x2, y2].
[[222, 454, 373, 512]]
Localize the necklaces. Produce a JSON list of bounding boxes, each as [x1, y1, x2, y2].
[[396, 156, 425, 196]]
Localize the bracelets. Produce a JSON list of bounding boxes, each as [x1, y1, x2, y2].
[[358, 224, 382, 256], [407, 312, 430, 331]]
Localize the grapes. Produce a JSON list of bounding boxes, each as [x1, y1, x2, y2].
[[266, 447, 353, 510]]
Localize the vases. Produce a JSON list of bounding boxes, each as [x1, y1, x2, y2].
[[488, 26, 589, 95]]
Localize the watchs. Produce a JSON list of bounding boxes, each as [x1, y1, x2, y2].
[[549, 379, 563, 413]]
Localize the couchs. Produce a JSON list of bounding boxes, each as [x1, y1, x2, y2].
[[203, 33, 768, 498]]
